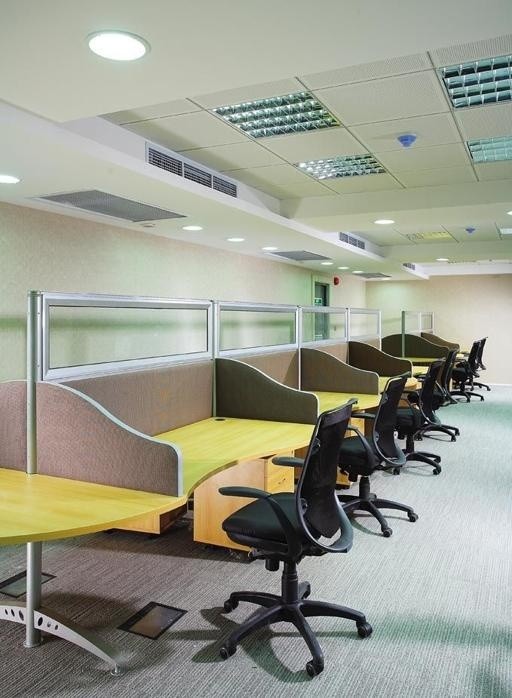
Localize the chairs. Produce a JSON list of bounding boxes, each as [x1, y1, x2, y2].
[[216, 396, 377, 643], [378, 334, 495, 474], [338, 378, 419, 539]]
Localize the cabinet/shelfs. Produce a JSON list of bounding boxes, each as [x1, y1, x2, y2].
[[295, 409, 367, 487], [193, 452, 296, 556]]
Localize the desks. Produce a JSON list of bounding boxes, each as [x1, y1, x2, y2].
[[302, 387, 379, 423], [150, 414, 315, 501], [1, 466, 179, 678]]
